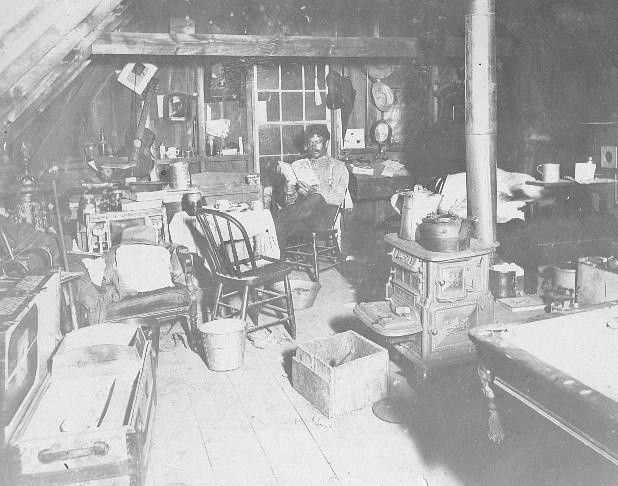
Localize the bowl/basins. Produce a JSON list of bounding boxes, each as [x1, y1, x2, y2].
[[268, 278, 321, 309]]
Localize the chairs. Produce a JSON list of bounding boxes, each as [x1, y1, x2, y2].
[[185, 205, 299, 342]]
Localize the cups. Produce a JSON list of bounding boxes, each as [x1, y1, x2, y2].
[[215, 200, 232, 212], [535, 163, 561, 184], [247, 175, 258, 186], [249, 200, 263, 211], [168, 147, 180, 159], [574, 162, 597, 183]]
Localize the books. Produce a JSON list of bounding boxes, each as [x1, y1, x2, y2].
[[278, 155, 320, 186]]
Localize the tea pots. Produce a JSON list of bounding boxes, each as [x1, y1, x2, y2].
[[390, 184, 479, 255]]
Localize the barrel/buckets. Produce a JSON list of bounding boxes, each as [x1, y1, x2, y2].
[[200, 319, 248, 370], [552, 260, 576, 289], [157, 162, 189, 191]]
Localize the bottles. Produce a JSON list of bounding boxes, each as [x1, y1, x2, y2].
[[98, 127, 108, 156], [101, 188, 122, 213]]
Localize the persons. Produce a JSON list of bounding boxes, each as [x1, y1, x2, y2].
[[273, 120, 349, 252]]
